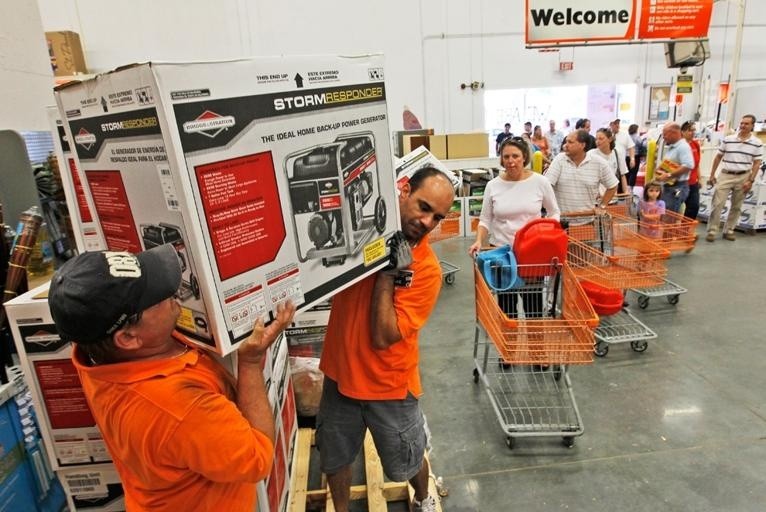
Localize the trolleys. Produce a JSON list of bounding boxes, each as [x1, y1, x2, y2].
[[279, 127, 387, 272], [609, 207, 699, 257], [135, 220, 200, 307], [471, 243, 601, 449], [564, 211, 670, 359], [598, 205, 686, 307], [439, 258, 460, 285]]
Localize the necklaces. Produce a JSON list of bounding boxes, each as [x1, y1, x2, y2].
[[89, 344, 188, 367]]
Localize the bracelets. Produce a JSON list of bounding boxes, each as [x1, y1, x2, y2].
[[748, 179, 756, 183]]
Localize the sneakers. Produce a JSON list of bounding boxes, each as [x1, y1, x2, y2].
[[706, 235, 715, 242], [721, 234, 735, 241], [499, 344, 517, 368], [410, 494, 436, 512], [528, 348, 550, 370]]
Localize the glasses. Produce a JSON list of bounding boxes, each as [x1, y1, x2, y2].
[[499, 137, 525, 145], [688, 129, 695, 131]]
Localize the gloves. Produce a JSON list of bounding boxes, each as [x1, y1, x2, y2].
[[379, 231, 412, 276]]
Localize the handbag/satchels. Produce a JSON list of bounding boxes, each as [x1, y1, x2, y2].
[[639, 145, 646, 155], [472, 244, 525, 291], [615, 168, 624, 201]]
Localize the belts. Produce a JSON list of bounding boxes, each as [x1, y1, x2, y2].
[[721, 169, 750, 174], [662, 181, 682, 187]]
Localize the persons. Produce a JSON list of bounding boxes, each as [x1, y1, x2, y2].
[[495, 119, 702, 239], [706, 115, 763, 242], [315, 167, 455, 511], [48, 242, 296, 512], [469, 137, 561, 371]]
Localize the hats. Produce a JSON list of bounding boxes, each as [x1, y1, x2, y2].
[[609, 118, 620, 125], [681, 120, 694, 130], [47, 243, 181, 343]]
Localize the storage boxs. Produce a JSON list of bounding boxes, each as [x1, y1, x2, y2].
[[698, 181, 766, 229], [394, 143, 461, 196], [465, 196, 483, 237], [52, 53, 402, 357], [438, 197, 463, 236], [47, 31, 86, 75], [285, 300, 334, 358]]
